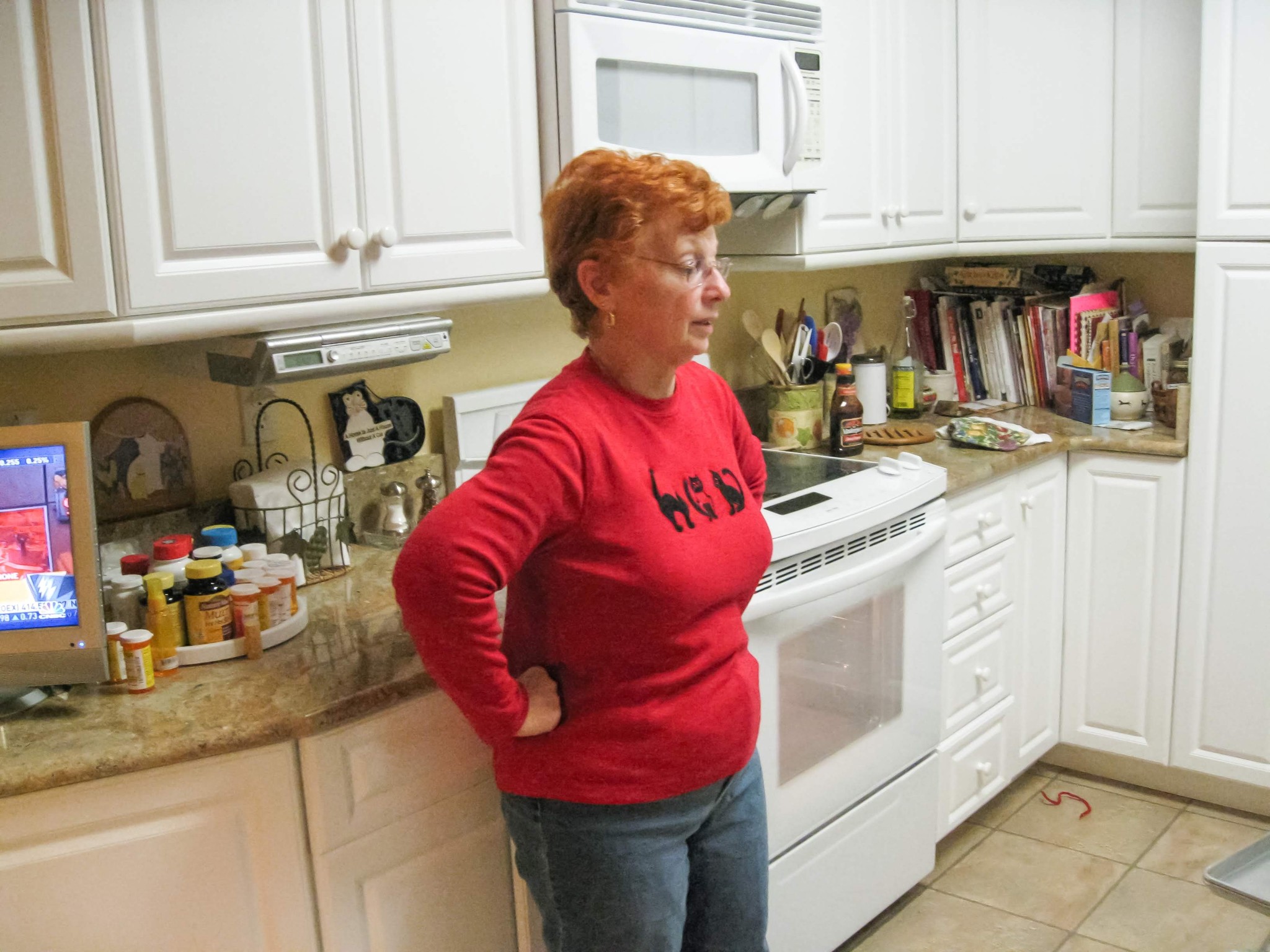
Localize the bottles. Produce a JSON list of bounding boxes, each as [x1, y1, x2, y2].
[[380, 481, 411, 536], [829, 362, 864, 458], [109, 523, 244, 648], [887, 295, 924, 419], [414, 466, 442, 525]]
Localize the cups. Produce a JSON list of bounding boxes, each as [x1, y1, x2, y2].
[[923, 370, 954, 413], [766, 379, 823, 450], [850, 353, 887, 426]]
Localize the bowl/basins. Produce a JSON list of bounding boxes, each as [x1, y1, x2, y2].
[[1110, 365, 1150, 420]]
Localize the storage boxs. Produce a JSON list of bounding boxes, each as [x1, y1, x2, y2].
[[1055, 355, 1113, 424]]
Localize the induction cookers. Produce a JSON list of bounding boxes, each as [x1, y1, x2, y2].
[[441, 353, 949, 566]]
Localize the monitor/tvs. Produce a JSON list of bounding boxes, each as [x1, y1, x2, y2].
[[0, 423, 112, 720]]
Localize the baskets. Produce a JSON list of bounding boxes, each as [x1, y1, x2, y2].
[[213, 398, 357, 588]]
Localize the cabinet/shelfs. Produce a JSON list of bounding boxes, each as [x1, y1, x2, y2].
[[0, 0, 554, 952], [714, 0, 1270, 850]]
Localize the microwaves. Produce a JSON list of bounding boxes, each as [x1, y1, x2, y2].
[[532, 0, 826, 210]]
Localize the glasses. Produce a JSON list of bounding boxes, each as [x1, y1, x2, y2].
[[568, 247, 731, 290]]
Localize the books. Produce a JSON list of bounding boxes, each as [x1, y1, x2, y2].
[[904, 264, 1184, 412]]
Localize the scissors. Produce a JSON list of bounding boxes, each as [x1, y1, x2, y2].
[[784, 358, 814, 385]]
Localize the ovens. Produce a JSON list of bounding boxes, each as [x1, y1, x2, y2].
[[742, 497, 949, 952]]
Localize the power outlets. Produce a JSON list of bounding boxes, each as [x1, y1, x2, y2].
[[236, 383, 278, 448], [1166, 317, 1193, 358]]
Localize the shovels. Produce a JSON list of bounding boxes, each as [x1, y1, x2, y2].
[[742, 309, 790, 380]]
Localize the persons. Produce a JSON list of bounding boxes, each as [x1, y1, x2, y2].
[[390, 148, 773, 951]]
